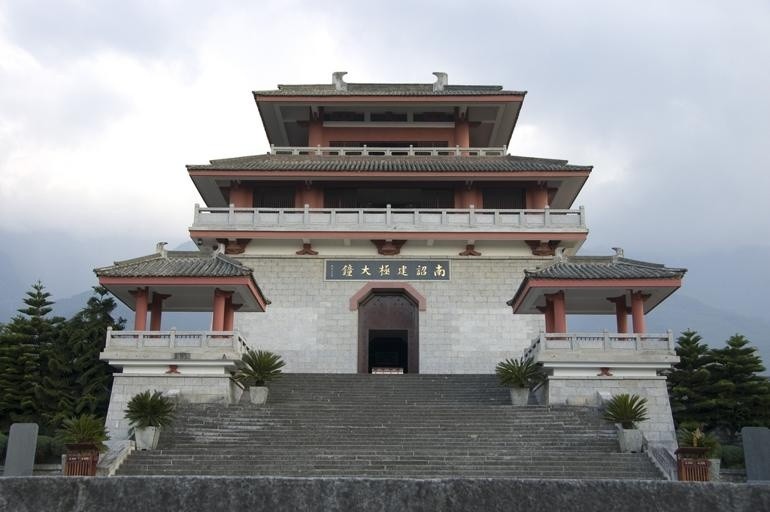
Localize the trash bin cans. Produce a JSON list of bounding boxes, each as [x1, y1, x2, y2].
[[674, 446, 712, 482], [63, 442, 99, 476]]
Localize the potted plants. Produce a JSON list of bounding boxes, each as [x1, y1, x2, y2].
[[599, 392, 651, 453], [231, 349, 287, 404], [494, 352, 550, 406], [678, 427, 723, 481], [123, 388, 178, 451], [51, 412, 112, 476]]
